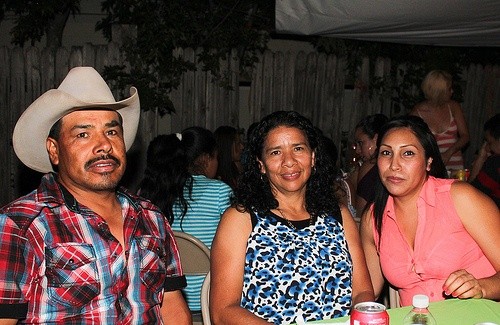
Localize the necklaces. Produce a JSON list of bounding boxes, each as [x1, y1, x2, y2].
[[277, 208, 316, 235]]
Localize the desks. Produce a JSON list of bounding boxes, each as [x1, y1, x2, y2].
[[290, 299, 500, 325]]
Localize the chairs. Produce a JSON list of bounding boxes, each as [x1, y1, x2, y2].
[[169, 230, 213, 325]]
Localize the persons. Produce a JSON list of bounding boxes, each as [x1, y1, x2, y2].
[[410, 69, 472, 181], [360, 115, 496, 324], [209, 109, 375, 324], [0, 66, 191, 325], [355, 114, 388, 218], [469, 111, 496, 196], [137, 127, 234, 325], [214, 125, 244, 188]]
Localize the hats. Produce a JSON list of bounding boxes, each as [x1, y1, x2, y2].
[[12, 66, 140, 174]]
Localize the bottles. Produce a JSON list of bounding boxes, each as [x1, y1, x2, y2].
[[403, 294, 437, 325]]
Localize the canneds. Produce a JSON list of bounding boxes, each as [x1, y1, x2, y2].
[[350, 301, 390, 325]]
